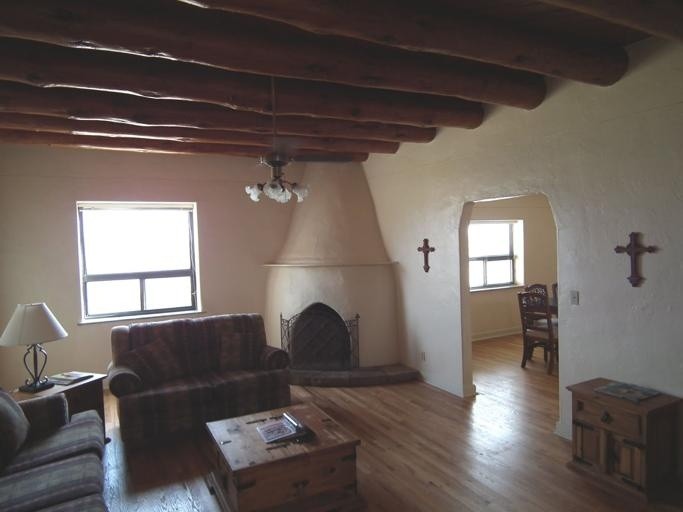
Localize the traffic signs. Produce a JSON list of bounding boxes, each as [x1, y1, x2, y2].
[[571, 292, 579, 303]]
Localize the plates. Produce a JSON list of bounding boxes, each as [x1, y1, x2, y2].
[[6, 371, 108, 423], [565, 378, 683, 503]]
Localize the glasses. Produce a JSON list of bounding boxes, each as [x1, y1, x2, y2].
[[244, 76, 308, 203], [0, 302, 68, 393]]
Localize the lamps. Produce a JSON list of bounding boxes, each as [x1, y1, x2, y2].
[[517, 283, 558, 375]]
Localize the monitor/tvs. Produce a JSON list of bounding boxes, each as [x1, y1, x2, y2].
[[49, 371, 93, 386]]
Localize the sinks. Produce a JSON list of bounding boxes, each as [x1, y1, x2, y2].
[[0, 388, 109, 512], [106, 313, 291, 441]]
